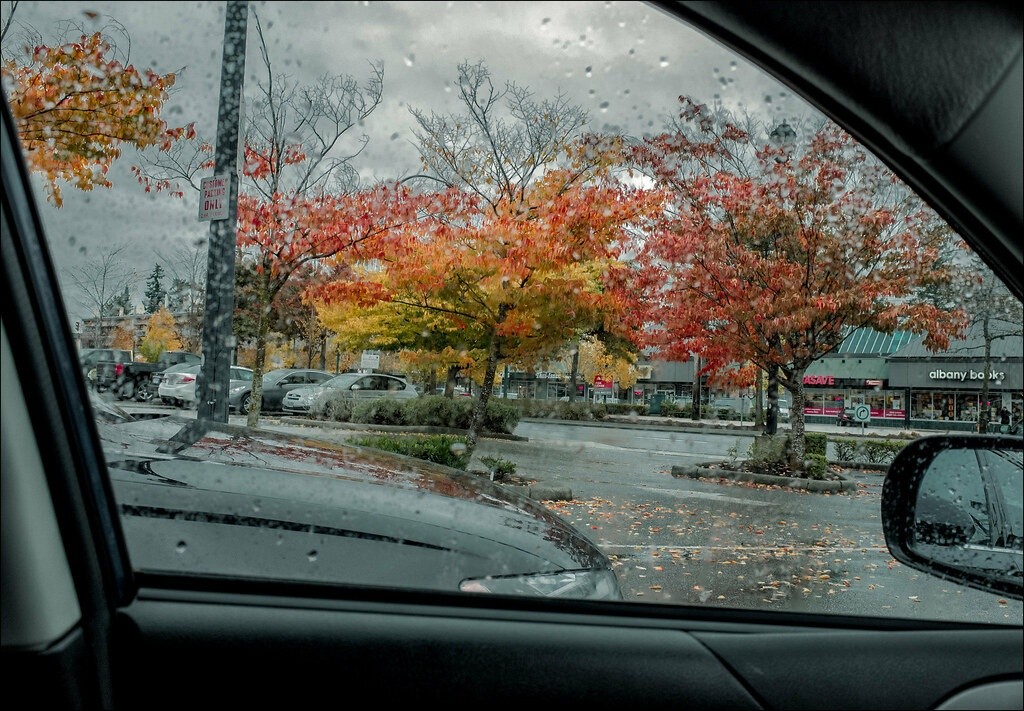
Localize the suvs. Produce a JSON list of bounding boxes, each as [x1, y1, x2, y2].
[[78, 348, 132, 387]]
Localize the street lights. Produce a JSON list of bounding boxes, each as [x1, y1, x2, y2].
[[334, 174, 454, 375]]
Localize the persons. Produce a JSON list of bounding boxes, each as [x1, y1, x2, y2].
[[370, 381, 377, 390], [1001, 407, 1012, 425]]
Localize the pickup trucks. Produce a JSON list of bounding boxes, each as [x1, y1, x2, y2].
[[96, 351, 201, 400]]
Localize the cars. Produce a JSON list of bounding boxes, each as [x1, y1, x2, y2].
[[281, 372, 418, 417], [714, 397, 737, 420], [559, 396, 590, 403], [158, 363, 271, 409], [151, 362, 200, 407], [762, 399, 791, 422], [89, 390, 624, 603], [229, 368, 342, 415], [836, 408, 867, 427], [427, 388, 470, 398]]
